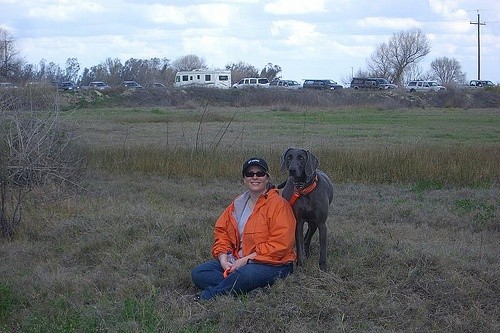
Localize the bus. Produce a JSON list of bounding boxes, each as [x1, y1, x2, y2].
[[173, 69, 231, 90]]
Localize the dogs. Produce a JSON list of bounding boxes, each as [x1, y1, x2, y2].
[[278, 148, 333, 272]]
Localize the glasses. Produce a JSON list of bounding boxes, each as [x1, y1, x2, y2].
[[244, 171, 268, 177]]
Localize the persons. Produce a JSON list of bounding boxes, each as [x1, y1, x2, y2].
[[191, 157, 297, 302]]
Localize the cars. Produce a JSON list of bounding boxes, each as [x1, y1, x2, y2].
[[0, 83, 20, 91], [25, 82, 39, 88], [150, 83, 164, 89], [62, 82, 79, 90], [121, 81, 142, 89]]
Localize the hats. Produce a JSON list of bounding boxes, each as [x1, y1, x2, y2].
[[243, 157, 268, 172]]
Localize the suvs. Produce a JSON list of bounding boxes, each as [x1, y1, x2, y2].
[[406, 81, 446, 94], [270, 79, 300, 91], [469, 80, 495, 94], [302, 79, 343, 91], [350, 78, 398, 90], [232, 77, 270, 89], [87, 82, 112, 92]]
